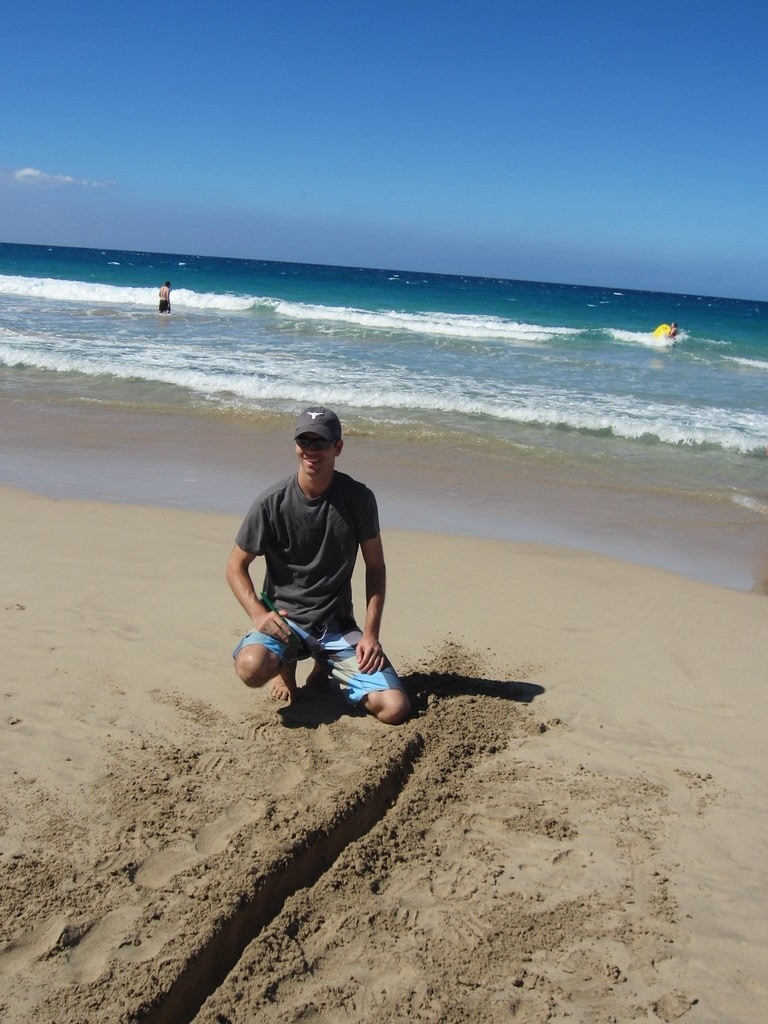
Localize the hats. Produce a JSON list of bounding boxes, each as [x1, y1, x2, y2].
[[293, 406, 342, 442]]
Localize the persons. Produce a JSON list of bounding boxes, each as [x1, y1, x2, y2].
[[666, 322, 678, 339], [224, 405, 412, 726], [158, 281, 172, 314]]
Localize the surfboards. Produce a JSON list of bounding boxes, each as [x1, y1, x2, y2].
[[652, 323, 672, 339]]
